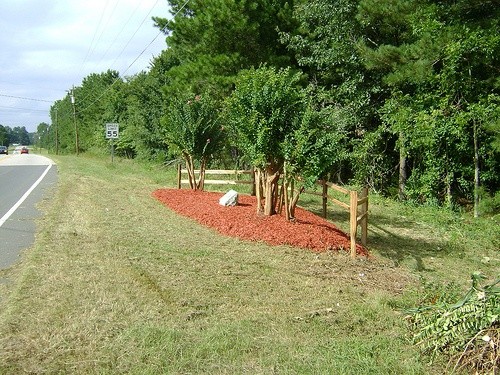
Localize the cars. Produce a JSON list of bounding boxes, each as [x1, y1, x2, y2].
[[20, 148, 28, 154], [0, 146, 8, 155]]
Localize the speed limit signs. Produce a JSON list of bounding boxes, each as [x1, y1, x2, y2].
[[106, 123, 119, 139]]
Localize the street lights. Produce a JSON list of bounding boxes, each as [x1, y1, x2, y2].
[[54, 107, 58, 157]]
